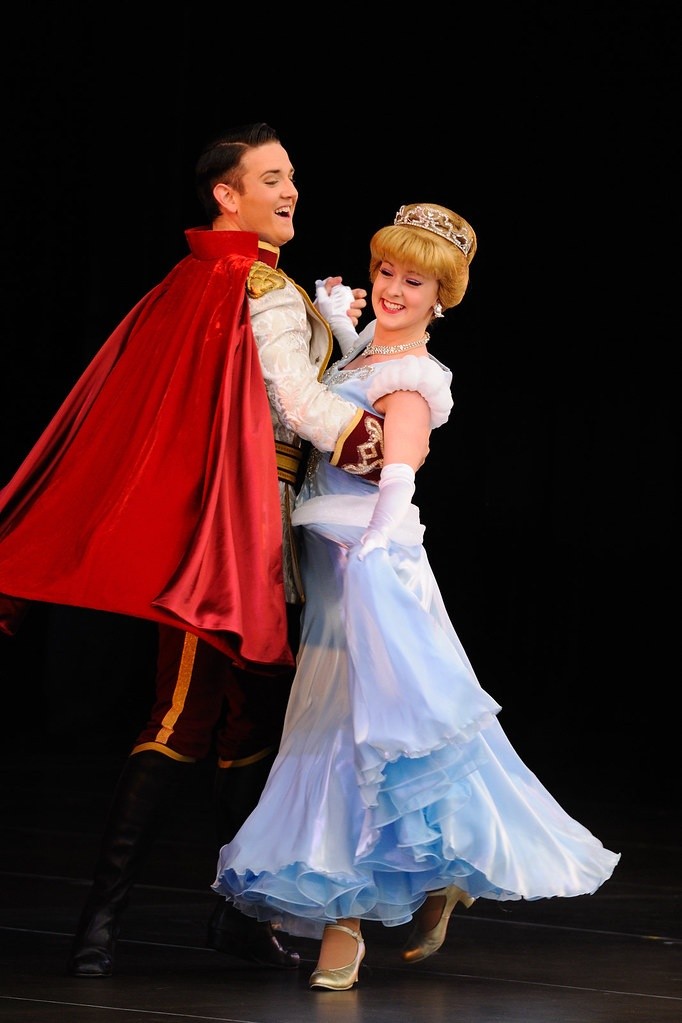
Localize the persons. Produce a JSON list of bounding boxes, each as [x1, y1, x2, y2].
[[210, 204, 620, 989], [0, 123, 430, 978]]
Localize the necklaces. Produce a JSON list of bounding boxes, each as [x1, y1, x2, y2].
[[362, 331, 430, 358]]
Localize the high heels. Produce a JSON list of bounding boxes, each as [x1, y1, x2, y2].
[[402, 880, 479, 964], [309, 924, 366, 990]]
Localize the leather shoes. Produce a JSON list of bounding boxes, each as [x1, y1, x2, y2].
[[75, 922, 112, 977], [217, 920, 300, 970]]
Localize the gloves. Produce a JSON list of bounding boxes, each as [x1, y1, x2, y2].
[[358, 462, 416, 556], [315, 277, 358, 358]]
